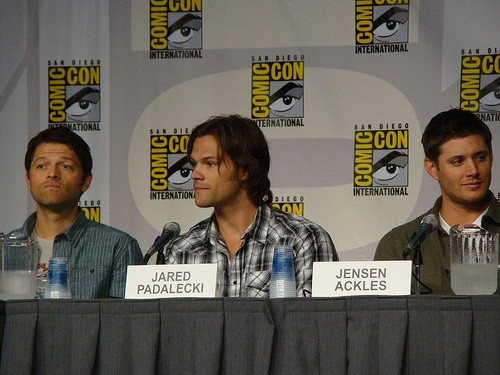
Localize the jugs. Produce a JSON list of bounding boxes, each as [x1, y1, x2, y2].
[[0, 232, 38, 300], [449, 224, 500, 295]]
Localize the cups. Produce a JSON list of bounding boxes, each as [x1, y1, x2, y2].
[[270, 245, 296, 298], [43, 256, 71, 299]]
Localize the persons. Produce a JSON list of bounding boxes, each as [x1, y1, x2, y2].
[[0, 126, 145, 300], [161, 115, 339, 297], [373, 106, 500, 296]]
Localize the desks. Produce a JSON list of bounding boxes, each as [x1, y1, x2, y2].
[[0, 294, 500, 375]]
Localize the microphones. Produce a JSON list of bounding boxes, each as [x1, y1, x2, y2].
[[145, 222, 182, 259], [403, 214, 439, 256]]
